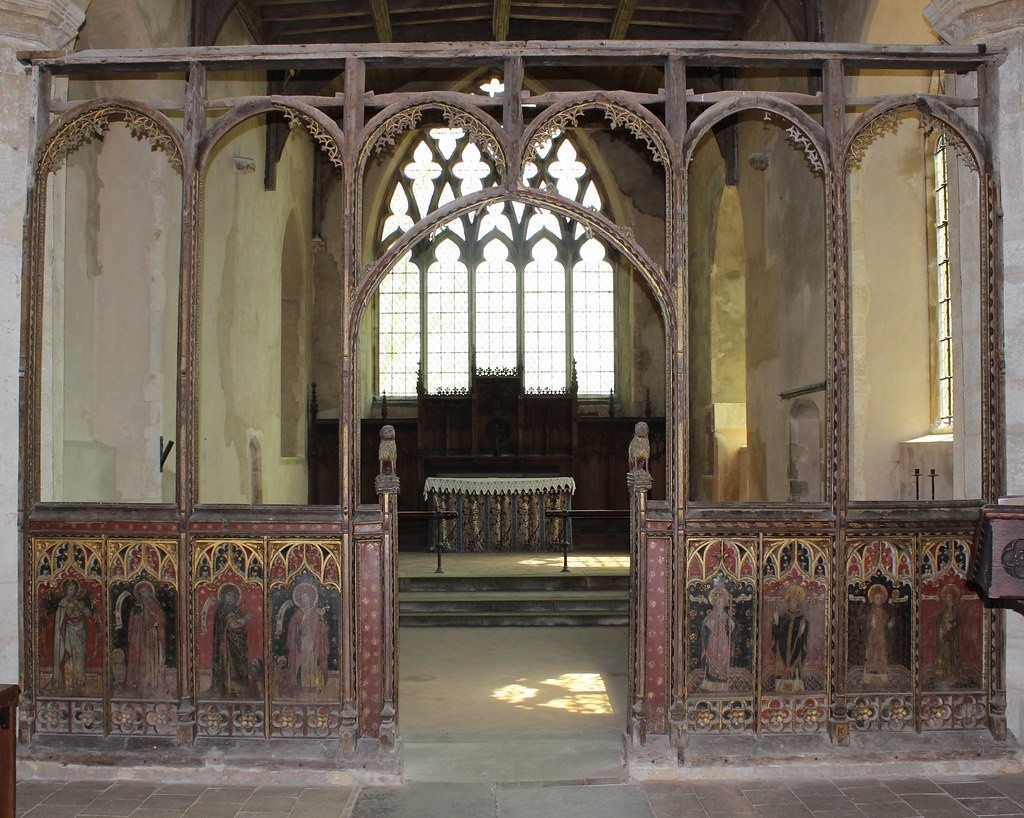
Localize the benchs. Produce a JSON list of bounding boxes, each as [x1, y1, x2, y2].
[[398, 511, 458, 573], [544, 509, 631, 571]]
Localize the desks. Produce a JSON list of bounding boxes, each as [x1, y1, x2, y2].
[[424, 477, 576, 552]]
